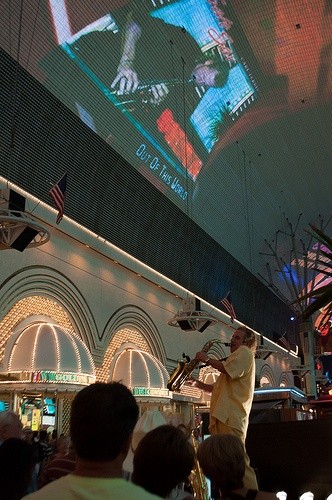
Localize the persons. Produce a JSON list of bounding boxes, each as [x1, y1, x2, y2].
[[184, 326, 259, 496], [43, 10, 228, 164], [0, 408, 202, 500], [129, 425, 193, 498], [194, 433, 283, 500], [20, 382, 168, 500]]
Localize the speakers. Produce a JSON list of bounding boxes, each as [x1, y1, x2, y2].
[[177, 311, 213, 333], [9, 223, 38, 253]]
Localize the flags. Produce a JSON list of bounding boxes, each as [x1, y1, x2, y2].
[[220, 289, 237, 321]]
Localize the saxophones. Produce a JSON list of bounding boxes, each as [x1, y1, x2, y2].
[[186, 404, 208, 500], [166, 338, 232, 391]]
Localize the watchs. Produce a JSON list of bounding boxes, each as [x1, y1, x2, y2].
[[204, 357, 210, 363]]
